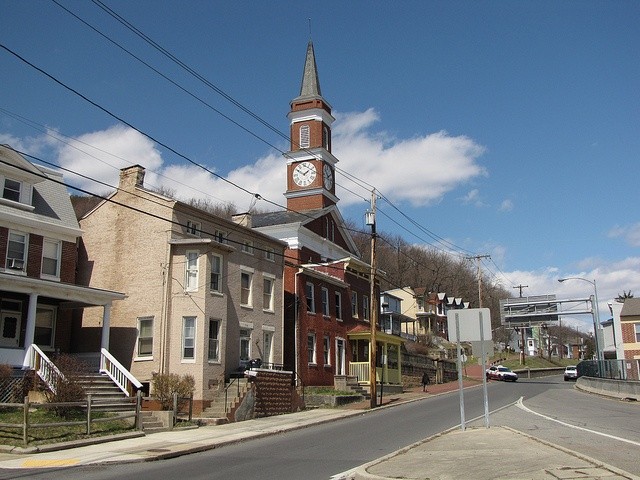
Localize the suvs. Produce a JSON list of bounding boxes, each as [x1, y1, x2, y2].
[[563, 365, 578, 381]]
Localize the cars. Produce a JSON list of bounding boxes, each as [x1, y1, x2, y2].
[[485, 365, 517, 382]]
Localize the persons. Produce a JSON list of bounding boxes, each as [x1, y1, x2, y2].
[[421, 372, 430, 391]]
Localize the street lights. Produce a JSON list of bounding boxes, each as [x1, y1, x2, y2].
[[558, 277, 602, 377]]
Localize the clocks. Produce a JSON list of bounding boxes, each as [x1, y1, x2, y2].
[[292, 162, 317, 187], [323, 164, 334, 191]]
